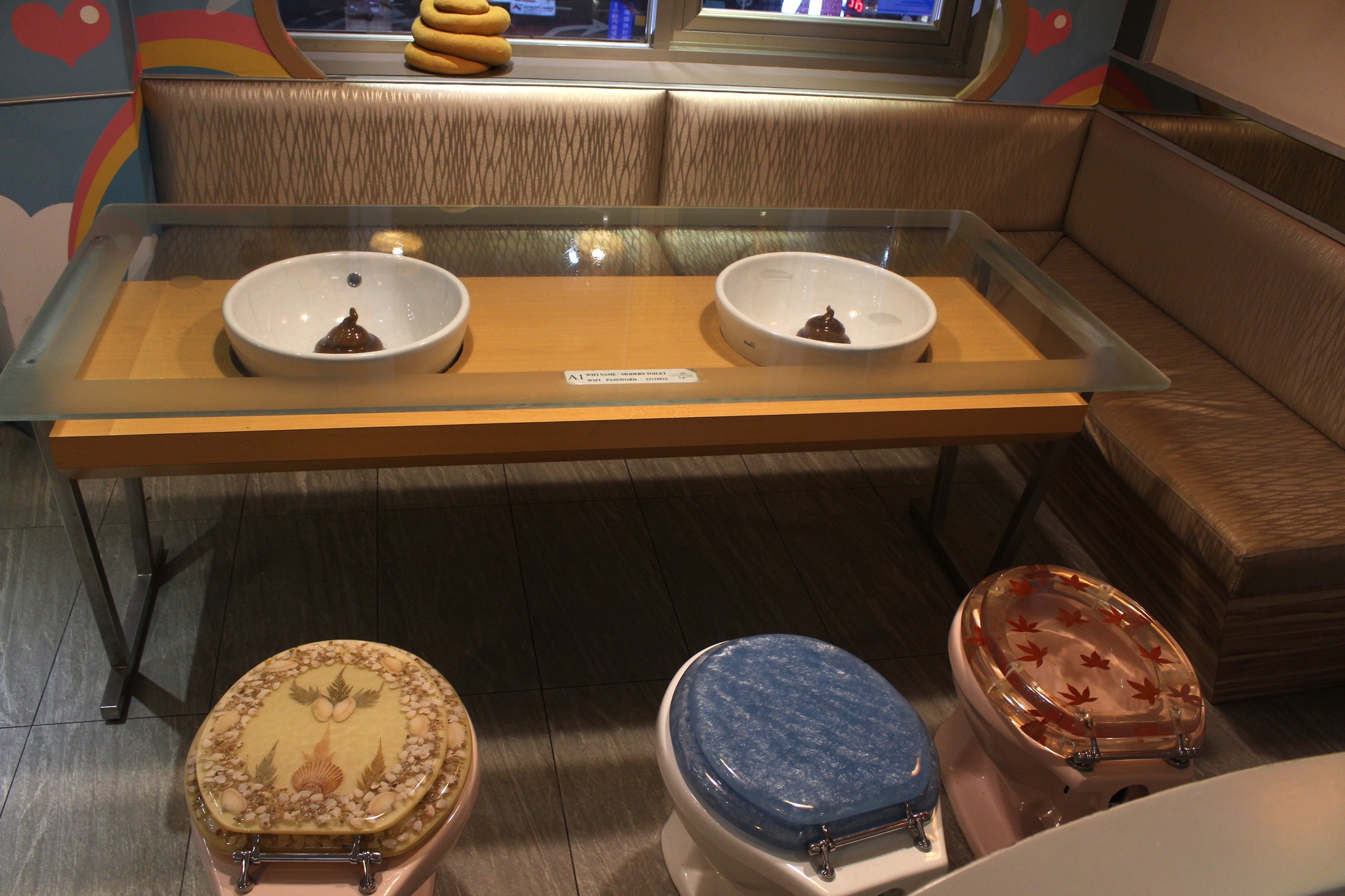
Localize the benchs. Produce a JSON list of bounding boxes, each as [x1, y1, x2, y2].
[[142, 79, 1344, 707]]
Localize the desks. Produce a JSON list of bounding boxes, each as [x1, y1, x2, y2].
[[0, 202, 1172, 724]]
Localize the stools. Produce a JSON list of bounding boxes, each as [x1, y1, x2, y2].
[[654, 635, 949, 896], [183, 638, 478, 896], [934, 566, 1206, 856]]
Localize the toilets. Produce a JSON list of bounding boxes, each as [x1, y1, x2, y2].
[[185, 640, 480, 896], [655, 634, 948, 896], [934, 565, 1206, 860]]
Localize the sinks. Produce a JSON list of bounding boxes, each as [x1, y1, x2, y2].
[[222, 250, 470, 377], [714, 251, 937, 367]]
